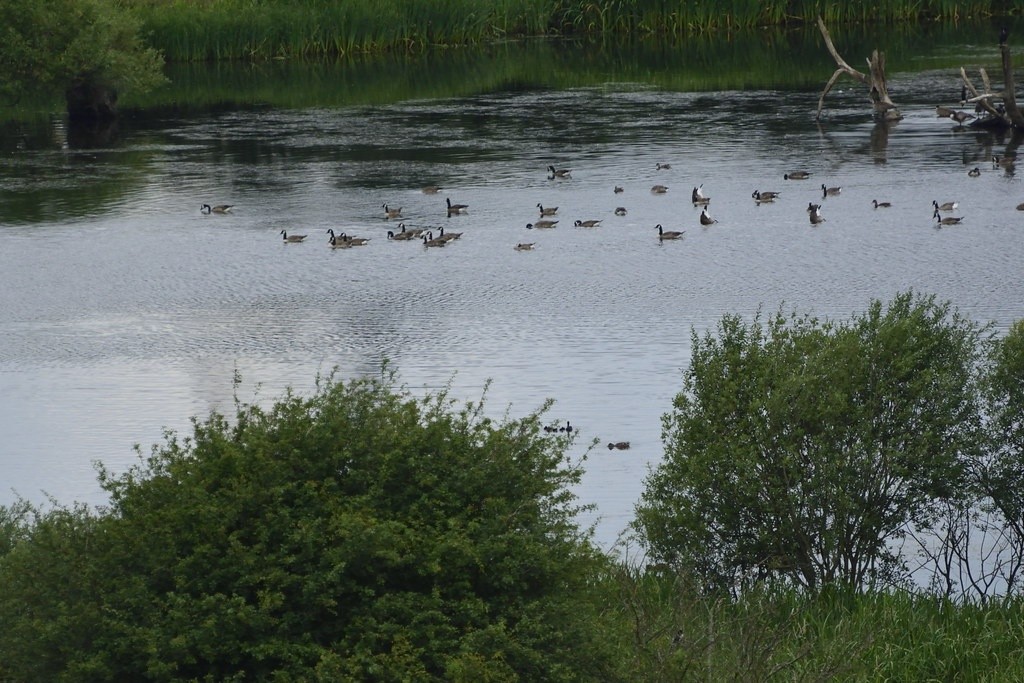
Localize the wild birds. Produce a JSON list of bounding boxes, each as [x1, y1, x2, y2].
[[604, 442, 635, 450], [198, 156, 1023, 255], [543, 420, 577, 437]]
